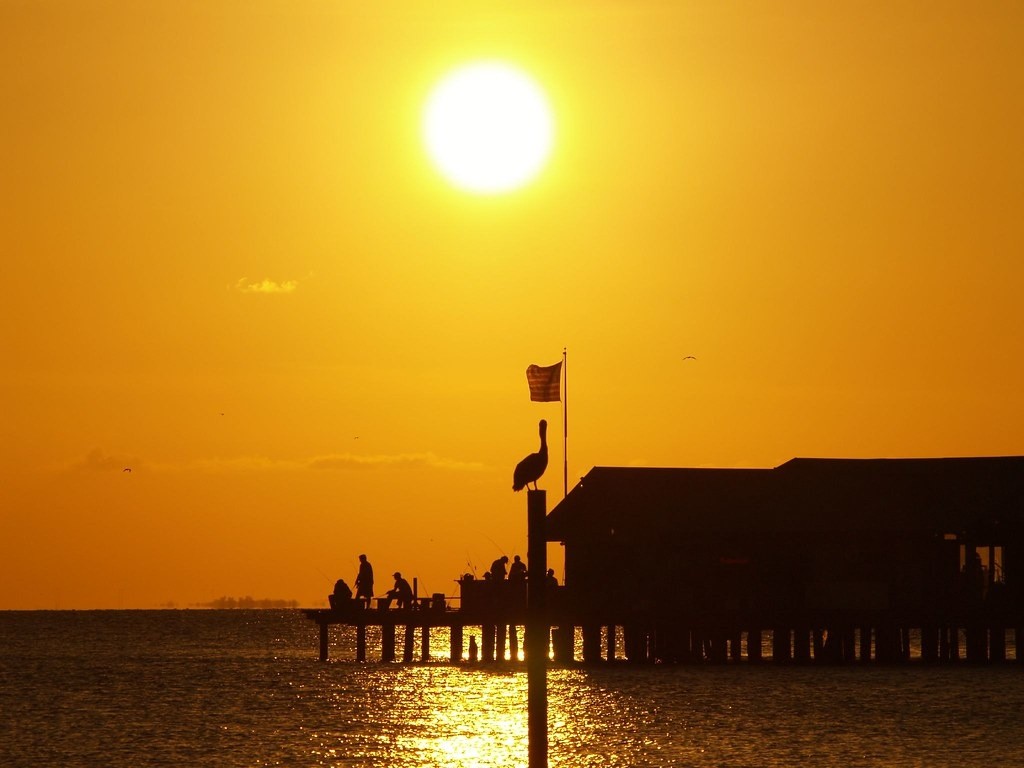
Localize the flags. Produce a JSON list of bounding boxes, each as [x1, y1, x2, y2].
[[526, 361, 562, 401]]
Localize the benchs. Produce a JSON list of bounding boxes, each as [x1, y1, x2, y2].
[[372, 597, 431, 610]]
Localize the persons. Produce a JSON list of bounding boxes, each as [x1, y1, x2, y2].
[[508, 555, 526, 579], [482, 556, 508, 580], [545, 569, 558, 587], [334, 579, 353, 599], [355, 555, 374, 609], [386, 572, 412, 608]]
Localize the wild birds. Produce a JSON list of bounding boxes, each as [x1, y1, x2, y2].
[[683, 356, 696, 360], [512, 419, 548, 493], [123, 468, 131, 472]]
